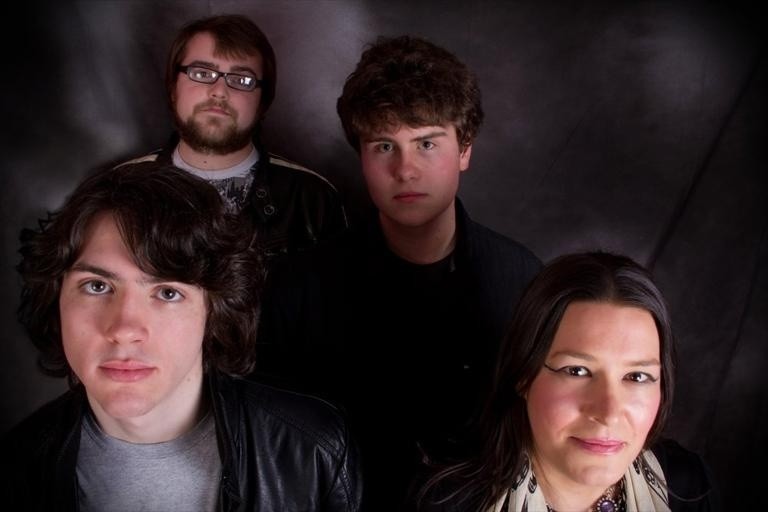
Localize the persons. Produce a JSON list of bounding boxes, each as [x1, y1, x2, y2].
[[415, 248, 710, 511], [336, 33, 547, 510], [107, 14, 356, 262], [0, 161, 365, 511]]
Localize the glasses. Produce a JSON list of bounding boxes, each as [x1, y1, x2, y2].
[[179, 64, 260, 93]]
